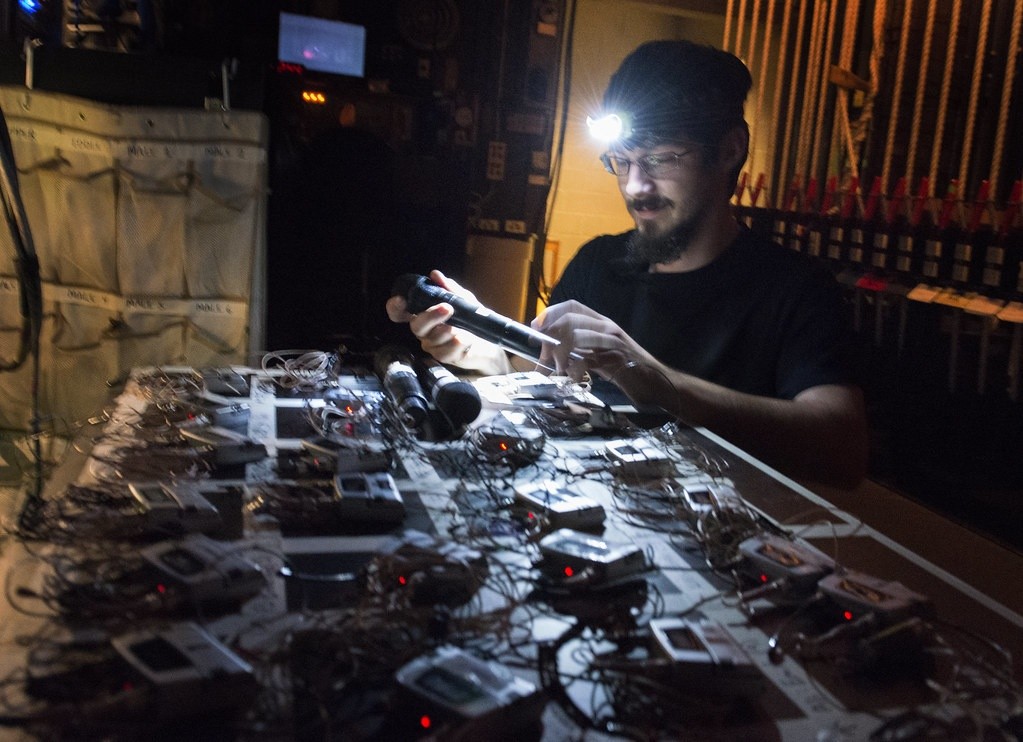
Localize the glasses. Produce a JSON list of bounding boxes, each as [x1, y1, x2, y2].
[[599, 145, 703, 179]]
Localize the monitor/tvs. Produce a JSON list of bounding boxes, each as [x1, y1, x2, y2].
[[277, 10, 368, 81]]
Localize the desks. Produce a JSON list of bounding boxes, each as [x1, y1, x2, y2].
[[12, 365, 1023, 742]]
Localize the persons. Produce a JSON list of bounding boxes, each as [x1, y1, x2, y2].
[[385, 40, 869, 478]]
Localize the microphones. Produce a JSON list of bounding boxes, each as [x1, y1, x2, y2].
[[415, 356, 481, 424], [391, 273, 583, 375], [376, 344, 430, 426]]
